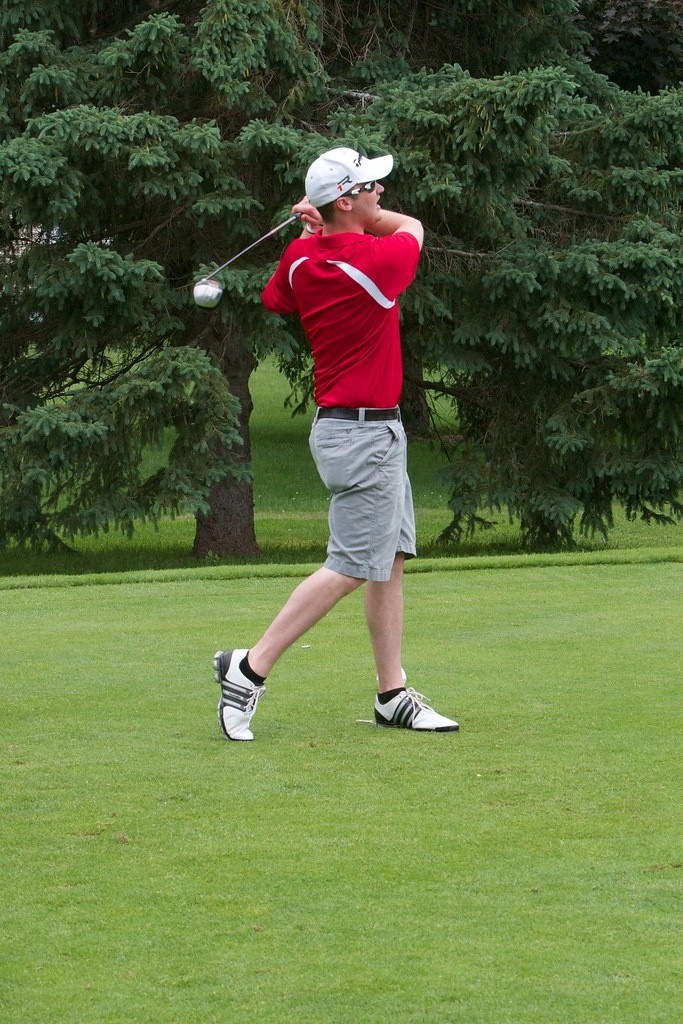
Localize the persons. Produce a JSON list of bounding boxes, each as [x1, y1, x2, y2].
[[212, 148, 460, 741]]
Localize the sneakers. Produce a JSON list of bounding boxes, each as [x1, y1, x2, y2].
[[212, 648, 265, 742], [375, 689, 460, 732]]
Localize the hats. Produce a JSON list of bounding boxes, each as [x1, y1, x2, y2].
[[304, 147, 394, 207]]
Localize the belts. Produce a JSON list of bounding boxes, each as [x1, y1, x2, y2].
[[317, 407, 399, 421]]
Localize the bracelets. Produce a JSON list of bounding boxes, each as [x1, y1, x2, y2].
[[304, 223, 317, 236]]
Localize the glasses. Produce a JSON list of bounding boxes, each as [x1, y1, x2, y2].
[[341, 181, 375, 197]]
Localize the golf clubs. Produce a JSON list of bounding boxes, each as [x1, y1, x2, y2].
[[193, 211, 303, 308]]
